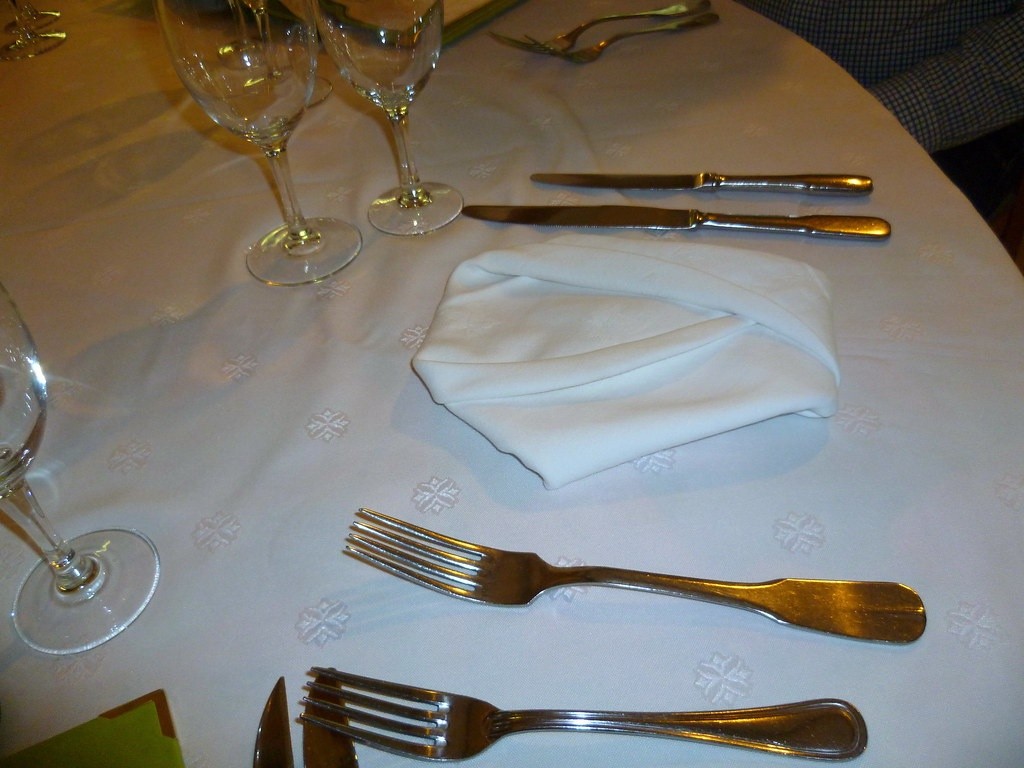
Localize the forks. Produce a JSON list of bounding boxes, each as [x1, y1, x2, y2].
[[485, 0, 711, 55], [300, 666, 868, 764], [524, 12, 720, 64], [345, 507, 927, 645]]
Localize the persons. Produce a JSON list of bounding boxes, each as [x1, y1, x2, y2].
[[737, 0, 1023, 221]]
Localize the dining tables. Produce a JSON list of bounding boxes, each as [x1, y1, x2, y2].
[[0, 0, 1024, 768]]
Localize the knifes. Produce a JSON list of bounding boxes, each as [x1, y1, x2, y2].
[[302, 668, 360, 768], [252, 676, 295, 768], [530, 172, 874, 197], [461, 204, 892, 243]]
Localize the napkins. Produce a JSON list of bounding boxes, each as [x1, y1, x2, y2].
[[411, 234, 839, 492]]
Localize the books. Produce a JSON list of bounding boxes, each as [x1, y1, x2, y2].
[[0, 692, 182, 766]]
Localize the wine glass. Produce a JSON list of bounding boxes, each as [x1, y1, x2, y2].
[[3, 0, 61, 36], [151, 0, 363, 285], [0, 0, 67, 62], [308, 0, 463, 234], [0, 285, 160, 655], [218, 0, 276, 70], [243, 0, 333, 111]]
